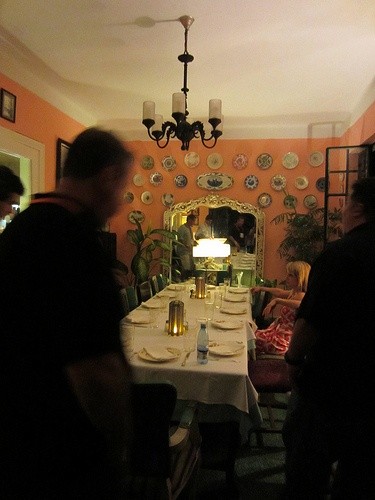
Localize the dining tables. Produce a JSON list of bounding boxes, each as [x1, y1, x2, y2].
[[120, 283, 260, 414]]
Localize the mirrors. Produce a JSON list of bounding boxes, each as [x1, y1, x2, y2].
[[162, 194, 266, 284]]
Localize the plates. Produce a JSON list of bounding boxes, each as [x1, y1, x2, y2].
[[221, 306, 248, 314], [212, 319, 242, 329], [123, 152, 259, 224], [208, 342, 245, 355], [256, 153, 272, 170], [139, 346, 181, 361], [309, 151, 325, 167], [281, 152, 299, 169], [303, 194, 317, 210], [228, 288, 248, 293], [146, 301, 166, 309], [168, 285, 184, 291], [284, 195, 297, 210], [224, 294, 247, 302], [126, 311, 155, 324], [294, 176, 309, 189], [270, 175, 286, 191], [258, 193, 273, 209], [158, 292, 177, 297], [316, 177, 330, 192]]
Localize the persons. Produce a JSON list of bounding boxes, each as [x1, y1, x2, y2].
[[0, 127, 134, 500], [250, 261, 311, 354], [112, 259, 129, 285], [175, 208, 254, 280], [281, 176, 375, 500], [0, 165, 25, 221]]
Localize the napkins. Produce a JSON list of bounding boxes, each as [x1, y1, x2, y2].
[[143, 345, 176, 360], [224, 294, 247, 301], [221, 308, 248, 313], [156, 291, 176, 297], [127, 311, 150, 323], [229, 287, 247, 293], [210, 341, 245, 354], [212, 319, 243, 328], [142, 301, 164, 308]]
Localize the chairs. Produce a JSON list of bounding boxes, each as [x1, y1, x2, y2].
[[62, 274, 295, 500]]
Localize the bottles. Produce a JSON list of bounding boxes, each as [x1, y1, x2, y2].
[[197, 324, 208, 365]]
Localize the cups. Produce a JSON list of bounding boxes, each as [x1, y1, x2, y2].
[[121, 324, 135, 348], [214, 292, 223, 308], [219, 283, 226, 300], [224, 278, 230, 291]]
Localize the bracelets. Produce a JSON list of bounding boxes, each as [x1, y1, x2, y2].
[[261, 287, 264, 291]]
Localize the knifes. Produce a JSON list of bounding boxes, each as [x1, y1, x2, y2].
[[182, 352, 190, 366]]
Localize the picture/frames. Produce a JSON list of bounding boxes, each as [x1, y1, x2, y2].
[[0, 88, 16, 123], [57, 138, 71, 187]]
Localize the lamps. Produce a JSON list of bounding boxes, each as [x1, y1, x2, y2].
[[142, 16, 224, 151]]
